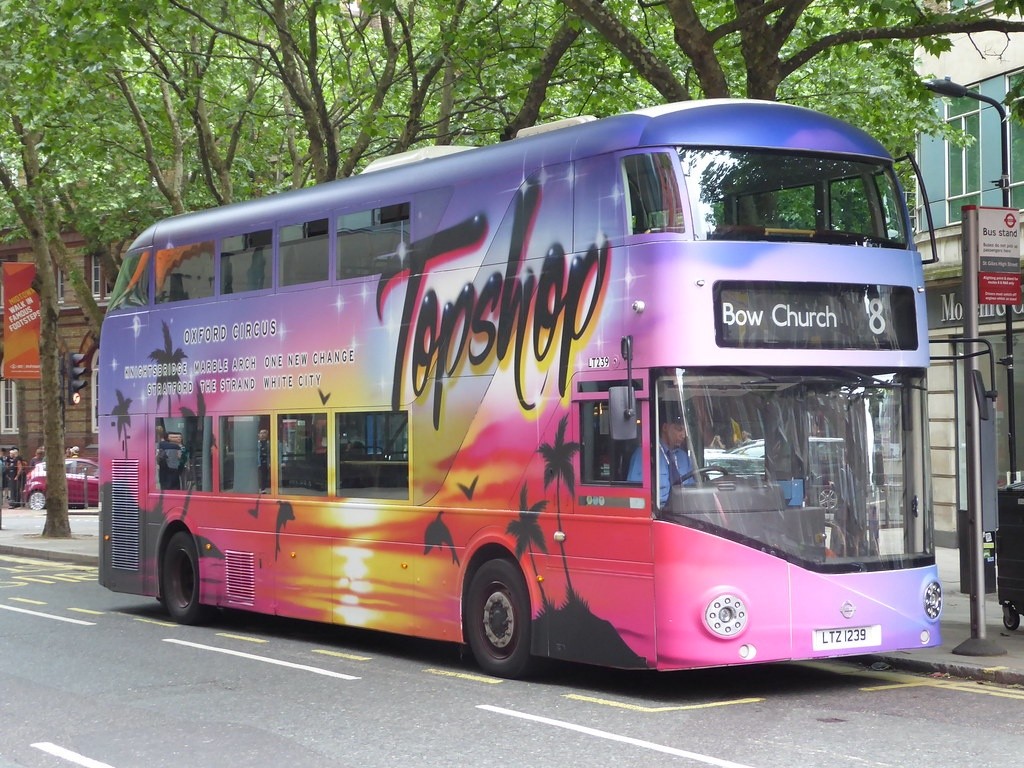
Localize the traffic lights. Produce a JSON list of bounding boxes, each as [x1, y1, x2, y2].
[[66, 351, 89, 405]]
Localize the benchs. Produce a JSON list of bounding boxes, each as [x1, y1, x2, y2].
[[387, 451, 408, 477], [349, 446, 384, 461]]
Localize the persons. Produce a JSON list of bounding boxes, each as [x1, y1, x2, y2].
[[0, 445, 79, 509], [155, 426, 189, 490], [625, 407, 696, 509], [257, 429, 287, 493]]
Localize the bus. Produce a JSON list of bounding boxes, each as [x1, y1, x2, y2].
[[98, 98, 999, 681]]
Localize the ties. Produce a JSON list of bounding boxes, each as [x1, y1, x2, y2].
[[669, 453, 680, 487]]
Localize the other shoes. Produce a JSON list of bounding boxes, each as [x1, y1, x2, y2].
[[9, 504, 15, 509]]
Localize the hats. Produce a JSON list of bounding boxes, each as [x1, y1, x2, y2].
[[70, 446, 80, 454], [662, 408, 688, 422], [36, 449, 42, 454]]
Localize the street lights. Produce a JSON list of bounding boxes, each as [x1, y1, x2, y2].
[[921, 78, 1018, 486]]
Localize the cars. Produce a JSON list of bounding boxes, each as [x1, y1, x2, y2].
[[22, 456, 98, 511]]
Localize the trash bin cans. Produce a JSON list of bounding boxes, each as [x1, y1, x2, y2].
[[996, 484, 1024, 614]]
